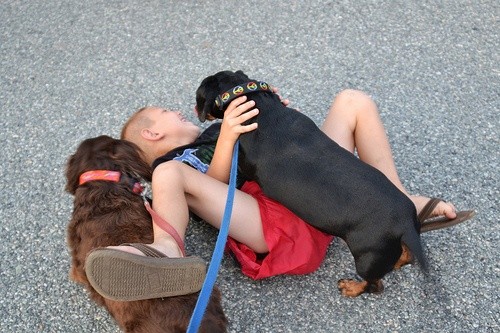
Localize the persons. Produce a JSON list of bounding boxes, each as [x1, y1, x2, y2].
[[85, 87, 474, 301]]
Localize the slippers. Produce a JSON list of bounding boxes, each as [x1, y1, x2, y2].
[[85, 242, 206, 301], [417, 197, 475, 233]]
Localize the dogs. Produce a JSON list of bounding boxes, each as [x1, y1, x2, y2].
[[195, 68, 430, 297], [62, 135, 230, 333]]
